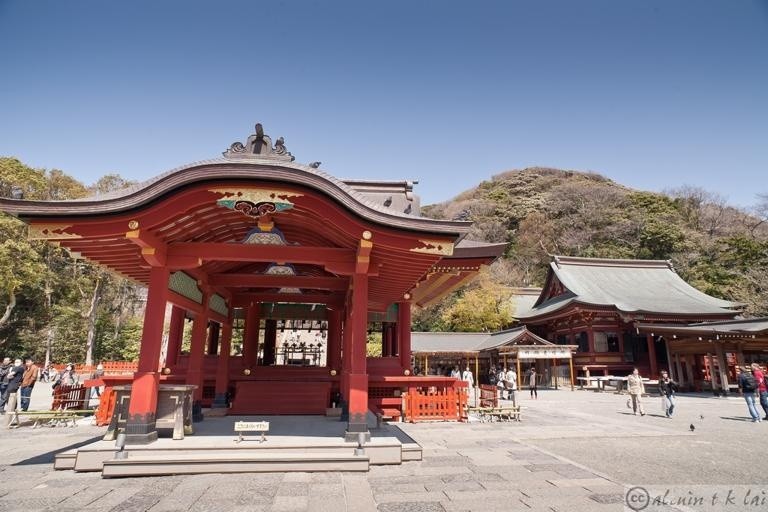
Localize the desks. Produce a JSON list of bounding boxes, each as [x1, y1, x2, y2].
[[103, 384, 199, 441]]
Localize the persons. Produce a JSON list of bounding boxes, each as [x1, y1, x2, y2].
[[527, 367, 538, 399], [0, 355, 59, 412], [625, 367, 646, 416], [657, 369, 678, 418], [415, 362, 518, 400], [51, 362, 76, 411], [87, 362, 106, 399], [749, 362, 768, 421], [737, 365, 763, 423]]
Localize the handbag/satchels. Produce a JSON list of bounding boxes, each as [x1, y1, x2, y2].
[[662, 397, 672, 410], [627, 399, 631, 409]]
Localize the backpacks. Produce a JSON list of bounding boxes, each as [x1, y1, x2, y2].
[[740, 372, 758, 391], [759, 370, 768, 387]]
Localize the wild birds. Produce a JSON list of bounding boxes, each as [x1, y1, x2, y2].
[[384, 196, 392, 207], [404, 204, 411, 214], [274, 137, 284, 146], [690, 424, 695, 431], [309, 162, 321, 168]]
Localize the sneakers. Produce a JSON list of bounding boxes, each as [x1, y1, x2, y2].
[[762, 415, 768, 420]]
